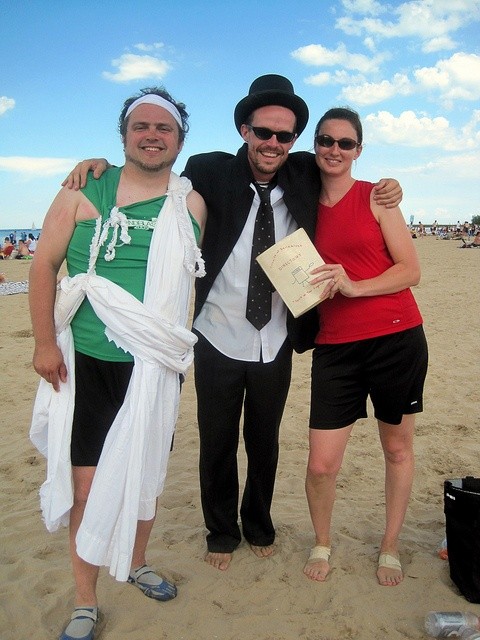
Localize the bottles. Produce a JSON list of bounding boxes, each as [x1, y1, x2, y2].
[[424, 611, 480, 636]]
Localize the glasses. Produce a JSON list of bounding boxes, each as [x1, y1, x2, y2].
[[316, 134, 360, 150], [247, 122, 295, 144]]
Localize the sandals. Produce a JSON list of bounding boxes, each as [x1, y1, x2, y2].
[[127, 563, 176, 600], [61, 608, 101, 640]]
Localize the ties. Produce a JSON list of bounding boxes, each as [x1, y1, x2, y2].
[[245, 175, 279, 331]]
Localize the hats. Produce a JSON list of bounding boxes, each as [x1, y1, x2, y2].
[[233, 74, 310, 138]]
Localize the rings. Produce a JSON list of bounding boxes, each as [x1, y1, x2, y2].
[[332, 279, 336, 285]]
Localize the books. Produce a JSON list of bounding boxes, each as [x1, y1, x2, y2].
[[254, 226, 336, 320]]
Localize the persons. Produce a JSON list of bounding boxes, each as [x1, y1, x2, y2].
[[408, 218, 480, 250], [0, 231, 39, 260], [178, 75, 322, 576], [29, 85, 207, 636], [303, 108, 428, 586]]
